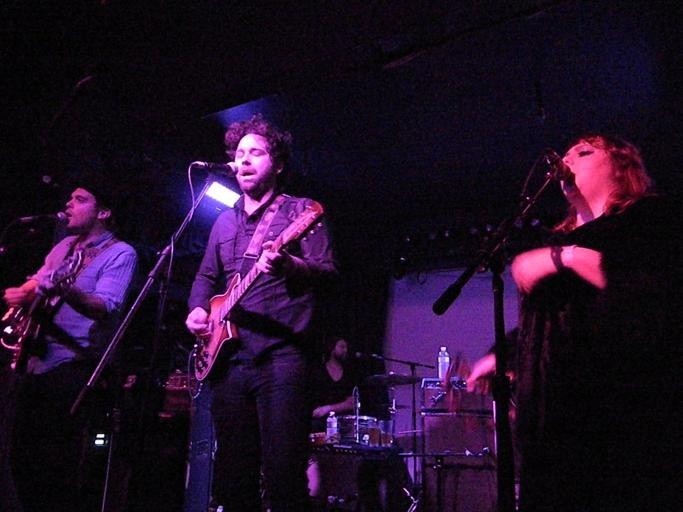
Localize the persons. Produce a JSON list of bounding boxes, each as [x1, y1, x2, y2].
[[466, 129, 683, 512], [3, 178, 140, 512], [183, 111, 340, 512], [308, 336, 358, 433]]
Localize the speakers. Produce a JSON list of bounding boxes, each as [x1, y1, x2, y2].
[[421, 412, 499, 512]]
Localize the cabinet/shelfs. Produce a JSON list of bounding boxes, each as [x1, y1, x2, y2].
[[421, 377, 517, 511]]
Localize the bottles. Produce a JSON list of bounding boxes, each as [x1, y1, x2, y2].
[[436, 345, 450, 379], [326, 411, 336, 439]]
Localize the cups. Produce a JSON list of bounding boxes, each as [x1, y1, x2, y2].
[[378, 420, 393, 447], [368, 420, 378, 446]]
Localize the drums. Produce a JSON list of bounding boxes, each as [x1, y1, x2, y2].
[[310, 415, 393, 447]]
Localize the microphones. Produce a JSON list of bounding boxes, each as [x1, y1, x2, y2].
[[481, 447, 489, 456], [20, 211, 68, 223], [355, 391, 361, 408], [546, 148, 571, 182], [195, 161, 239, 177], [355, 351, 378, 358]]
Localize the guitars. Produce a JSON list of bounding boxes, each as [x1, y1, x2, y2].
[[193, 201, 323, 381], [11, 249, 82, 371]]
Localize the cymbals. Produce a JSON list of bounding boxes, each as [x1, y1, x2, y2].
[[370, 375, 421, 385]]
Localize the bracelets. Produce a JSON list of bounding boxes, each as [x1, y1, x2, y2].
[[551, 243, 563, 272]]
